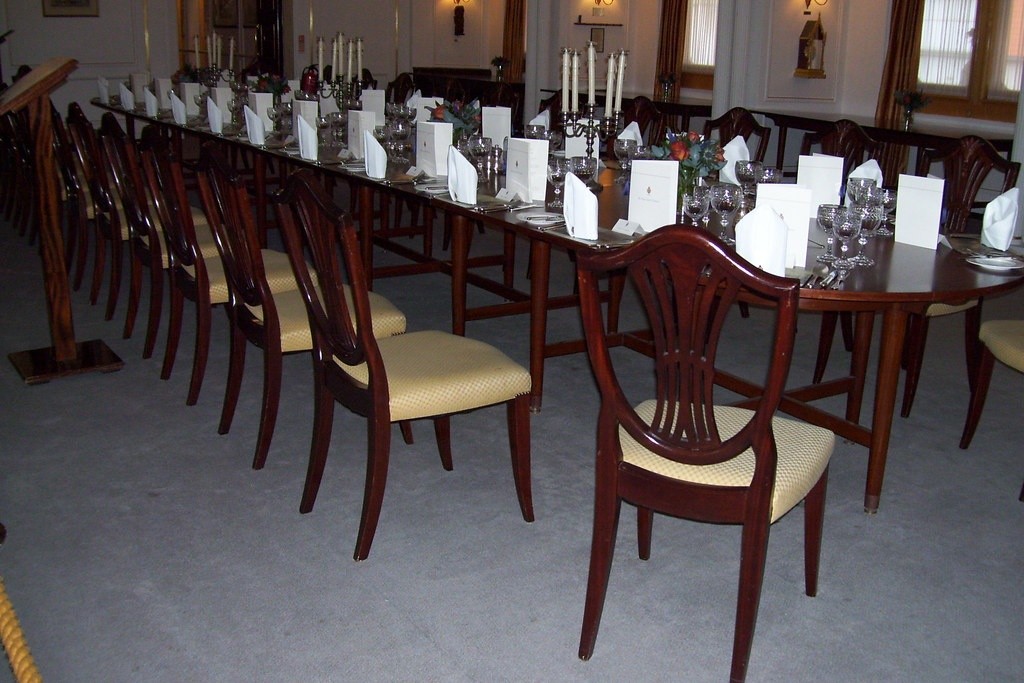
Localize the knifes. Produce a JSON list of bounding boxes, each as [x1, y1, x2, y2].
[[820, 270, 838, 287]]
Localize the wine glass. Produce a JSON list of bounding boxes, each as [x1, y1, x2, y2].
[[547, 156, 597, 208], [817, 177, 897, 269], [614, 139, 654, 183], [684, 161, 783, 246], [316, 90, 362, 147], [226, 81, 251, 125], [195, 92, 212, 118], [373, 103, 417, 164], [457, 136, 492, 183], [267, 91, 318, 144]]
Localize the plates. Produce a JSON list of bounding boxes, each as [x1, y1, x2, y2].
[[338, 164, 365, 171], [516, 212, 564, 225], [198, 126, 211, 131], [414, 184, 449, 194], [236, 136, 249, 141], [965, 257, 1023, 270], [280, 147, 300, 155]]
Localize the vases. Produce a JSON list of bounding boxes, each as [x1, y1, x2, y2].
[[677, 158, 696, 215], [901, 113, 914, 133], [274, 93, 281, 111], [496, 70, 504, 85], [661, 84, 675, 104]]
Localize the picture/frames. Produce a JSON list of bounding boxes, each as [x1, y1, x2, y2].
[[42, 0, 100, 18]]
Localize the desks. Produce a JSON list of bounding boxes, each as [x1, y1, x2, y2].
[[749, 109, 1017, 209], [407, 71, 525, 136], [85, 90, 1024, 517], [538, 88, 713, 132]]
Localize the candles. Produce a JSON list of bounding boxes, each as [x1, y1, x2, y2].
[[192, 30, 235, 70], [561, 40, 629, 119], [317, 31, 363, 86]]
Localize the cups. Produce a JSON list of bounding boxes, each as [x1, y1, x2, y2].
[[167, 88, 180, 100], [523, 125, 563, 150]]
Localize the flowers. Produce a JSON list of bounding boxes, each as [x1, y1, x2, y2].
[[650, 124, 727, 177], [168, 64, 198, 83], [490, 54, 512, 67], [248, 71, 292, 94], [658, 71, 678, 83], [424, 97, 481, 131], [895, 84, 934, 108]]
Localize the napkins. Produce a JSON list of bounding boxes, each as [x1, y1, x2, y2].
[[120, 83, 134, 111], [207, 96, 223, 134], [445, 110, 1020, 279], [363, 129, 388, 178], [407, 89, 422, 124], [169, 93, 187, 125], [243, 105, 264, 145], [96, 79, 109, 105], [144, 87, 159, 118], [297, 115, 318, 162]]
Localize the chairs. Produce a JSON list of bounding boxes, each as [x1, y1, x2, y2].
[[0, 64, 1024, 683], [316, 65, 587, 139]]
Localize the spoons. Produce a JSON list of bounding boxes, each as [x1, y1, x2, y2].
[[807, 264, 829, 288]]
